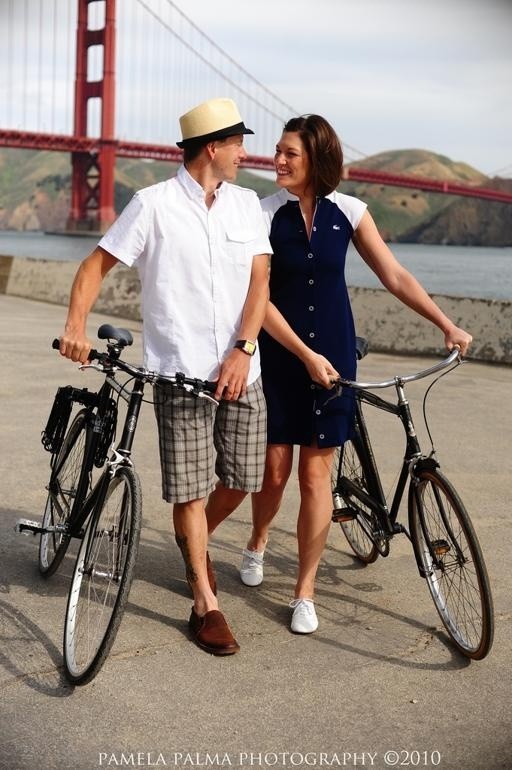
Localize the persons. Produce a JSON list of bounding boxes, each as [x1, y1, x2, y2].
[[57, 95, 276, 654], [238, 111, 476, 635]]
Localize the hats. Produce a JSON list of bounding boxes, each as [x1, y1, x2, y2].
[[177, 98, 254, 148]]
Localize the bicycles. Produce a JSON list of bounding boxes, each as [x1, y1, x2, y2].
[[12, 324, 227, 686], [327, 338, 494, 660]]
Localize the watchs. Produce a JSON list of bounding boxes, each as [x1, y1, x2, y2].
[[232, 338, 258, 356]]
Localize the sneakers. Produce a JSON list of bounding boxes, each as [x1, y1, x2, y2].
[[207, 550, 217, 594], [241, 547, 264, 587], [289, 598, 318, 634], [187, 606, 239, 655]]
